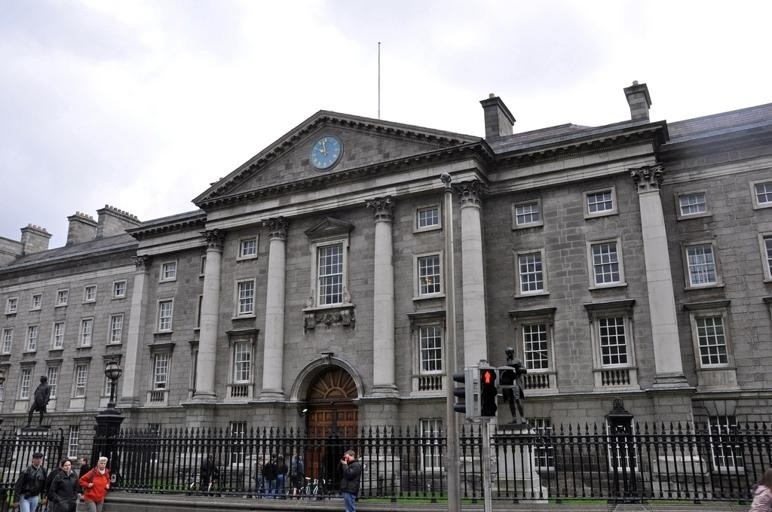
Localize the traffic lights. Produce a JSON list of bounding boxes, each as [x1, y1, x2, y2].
[[453, 365, 516, 423]]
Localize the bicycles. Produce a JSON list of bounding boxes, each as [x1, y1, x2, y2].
[[286, 477, 325, 501], [190, 472, 226, 498]]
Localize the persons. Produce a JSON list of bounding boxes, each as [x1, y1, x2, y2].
[[502, 347, 527, 425], [13, 453, 111, 512], [252, 450, 304, 499], [340, 450, 361, 512], [747, 469, 772, 512], [25, 376, 50, 430], [201, 455, 220, 496]]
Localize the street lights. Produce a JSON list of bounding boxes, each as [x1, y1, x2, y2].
[[90, 356, 127, 472]]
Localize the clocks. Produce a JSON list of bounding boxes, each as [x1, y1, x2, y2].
[[309, 135, 343, 172]]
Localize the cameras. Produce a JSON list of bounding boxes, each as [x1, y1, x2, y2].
[[342, 457, 345, 461]]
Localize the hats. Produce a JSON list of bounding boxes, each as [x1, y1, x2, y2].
[[32, 453, 43, 459]]
